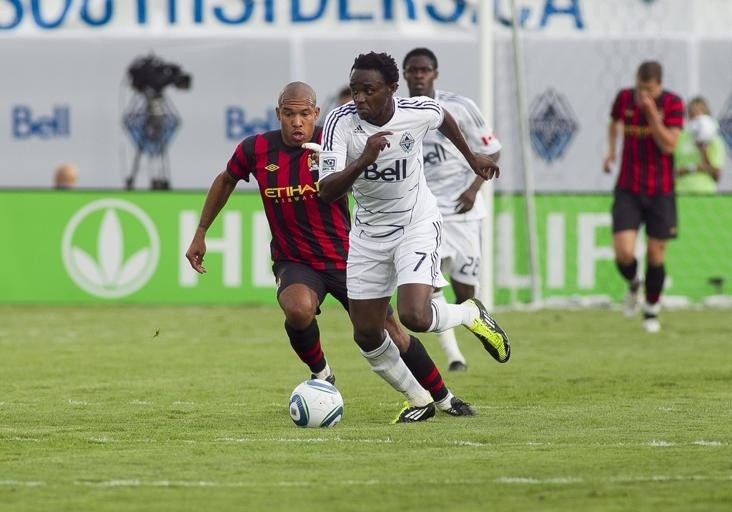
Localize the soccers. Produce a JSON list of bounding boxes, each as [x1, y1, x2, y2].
[[287, 378, 345, 429]]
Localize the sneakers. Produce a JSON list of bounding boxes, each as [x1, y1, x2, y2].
[[440, 394, 478, 416], [447, 361, 467, 371], [624, 274, 645, 319], [389, 401, 436, 423], [310, 372, 335, 385], [461, 297, 511, 363], [642, 301, 662, 333]]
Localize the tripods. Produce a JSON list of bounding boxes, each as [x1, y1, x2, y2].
[[122, 89, 174, 190]]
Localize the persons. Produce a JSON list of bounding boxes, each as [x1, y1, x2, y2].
[[186, 81, 478, 419], [676, 109, 732, 194], [601, 59, 687, 334], [332, 88, 354, 105], [316, 50, 511, 426], [675, 96, 719, 151], [401, 46, 503, 374], [53, 164, 77, 189]]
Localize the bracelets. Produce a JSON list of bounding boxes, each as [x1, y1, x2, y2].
[[685, 164, 697, 175], [197, 223, 209, 229]]
